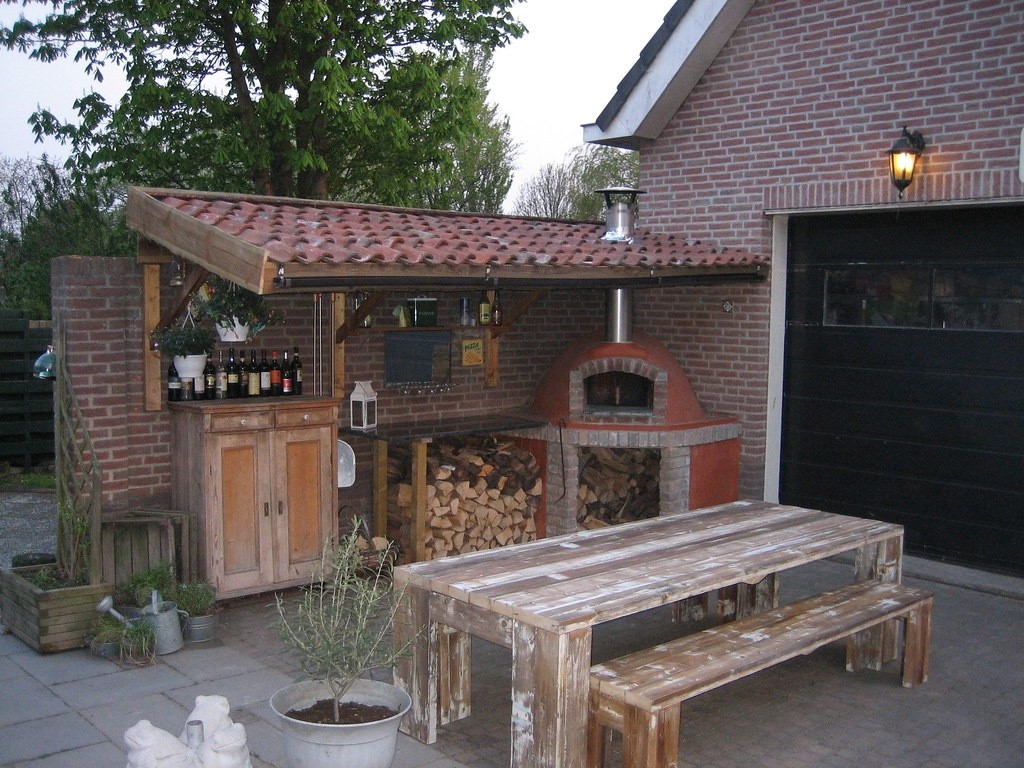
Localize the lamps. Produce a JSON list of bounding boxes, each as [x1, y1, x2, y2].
[[884, 124, 924, 200]]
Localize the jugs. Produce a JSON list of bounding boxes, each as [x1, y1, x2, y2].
[[94, 594, 190, 656]]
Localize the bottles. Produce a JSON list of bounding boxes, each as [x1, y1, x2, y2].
[[194, 372, 205, 400], [168, 361, 180, 401], [247, 349, 260, 397], [349, 380, 378, 429], [216, 350, 227, 398], [362, 291, 372, 327], [491, 290, 502, 326], [226, 348, 238, 399], [270, 350, 281, 396], [291, 347, 302, 394], [281, 349, 291, 395], [180, 376, 194, 400], [259, 349, 271, 396], [204, 352, 216, 399], [478, 290, 491, 325], [237, 350, 248, 397], [352, 298, 362, 327]]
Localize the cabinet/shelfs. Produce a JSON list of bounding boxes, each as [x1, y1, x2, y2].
[[172, 397, 343, 607]]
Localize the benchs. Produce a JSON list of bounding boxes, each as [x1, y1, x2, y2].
[[587, 577, 936, 768]]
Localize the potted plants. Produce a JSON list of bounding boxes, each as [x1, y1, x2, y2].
[[84, 564, 218, 662], [155, 323, 214, 379], [268, 518, 428, 768], [196, 275, 286, 341]]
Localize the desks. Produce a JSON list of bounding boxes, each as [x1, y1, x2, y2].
[[392, 498, 904, 768], [338, 415, 544, 566]]
